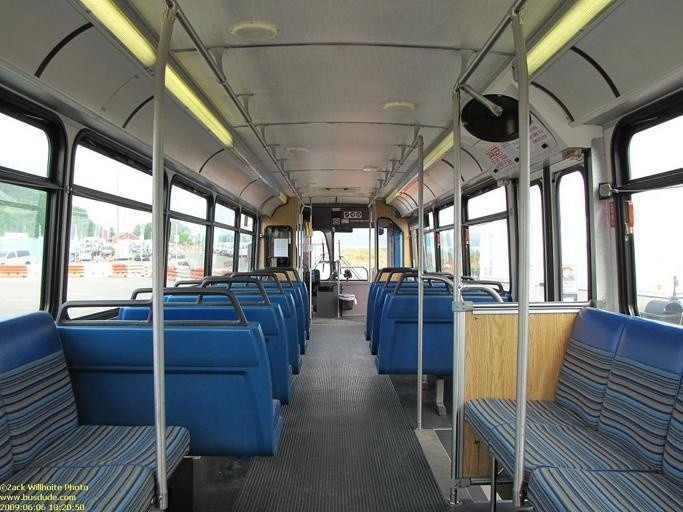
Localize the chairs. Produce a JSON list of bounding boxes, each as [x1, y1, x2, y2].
[[462, 306, 682, 509], [0, 312, 191, 512]]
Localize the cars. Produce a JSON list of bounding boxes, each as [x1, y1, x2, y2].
[[1, 231, 251, 271]]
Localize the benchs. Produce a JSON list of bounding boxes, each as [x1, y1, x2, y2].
[[362, 267, 514, 417], [56, 268, 311, 458]]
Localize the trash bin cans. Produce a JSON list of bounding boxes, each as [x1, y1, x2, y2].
[[338, 293, 356, 310]]
